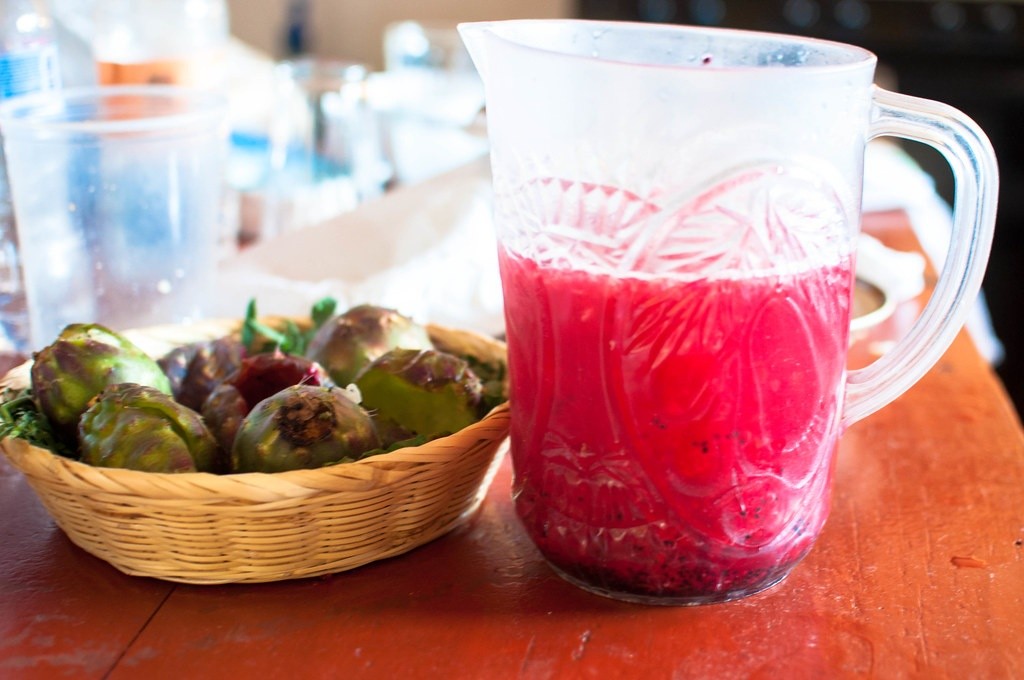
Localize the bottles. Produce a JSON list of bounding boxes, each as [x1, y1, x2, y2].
[[0, 0, 108, 294]]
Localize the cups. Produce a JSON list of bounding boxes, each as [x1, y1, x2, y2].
[[457, 20, 1001, 606], [0, 86, 220, 348]]
